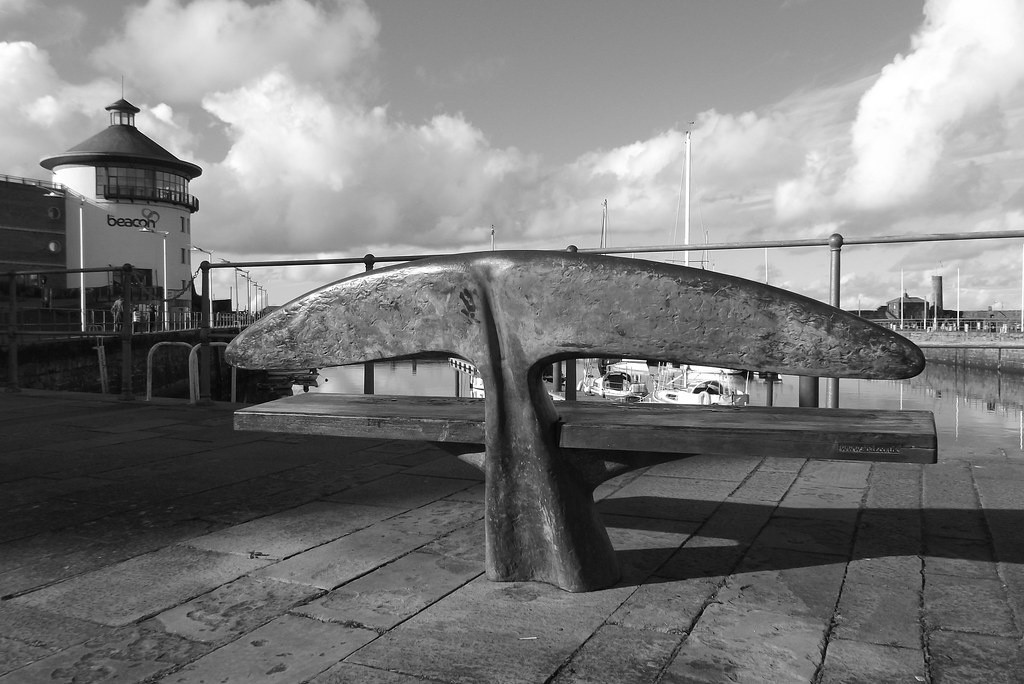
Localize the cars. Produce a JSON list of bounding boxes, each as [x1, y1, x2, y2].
[[0, 269, 47, 298]]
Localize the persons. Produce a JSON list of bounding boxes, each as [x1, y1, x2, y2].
[[110, 294, 127, 332], [133, 307, 140, 332], [146, 303, 157, 331]]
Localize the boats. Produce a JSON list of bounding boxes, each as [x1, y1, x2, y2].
[[650, 361, 751, 407], [582, 358, 648, 403]]
